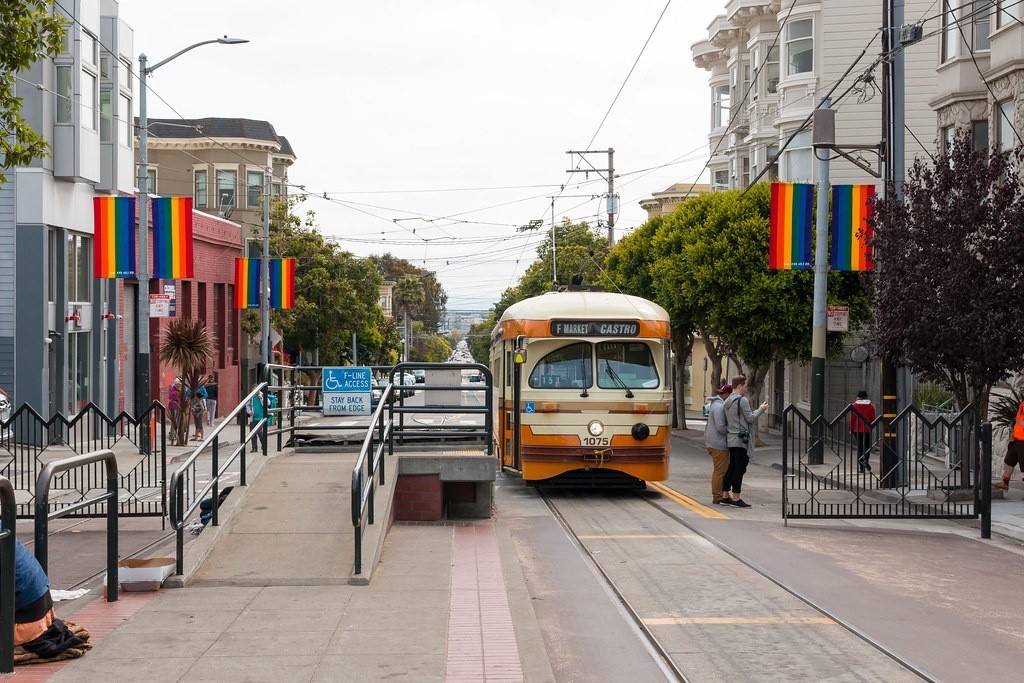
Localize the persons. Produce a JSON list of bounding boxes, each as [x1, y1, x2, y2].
[[246, 384, 271, 452], [991, 400, 1024, 501], [719, 374, 769, 508], [848, 390, 876, 473], [168, 375, 218, 443], [15, 536, 56, 653], [704, 384, 733, 503]]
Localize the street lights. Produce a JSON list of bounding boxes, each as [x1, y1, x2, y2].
[[133, 34, 250, 456]]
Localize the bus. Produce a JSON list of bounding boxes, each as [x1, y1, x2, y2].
[[488, 281, 672, 496]]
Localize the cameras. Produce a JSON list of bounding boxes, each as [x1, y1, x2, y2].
[[738, 431, 749, 444]]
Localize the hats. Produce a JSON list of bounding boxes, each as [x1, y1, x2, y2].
[[716, 384, 732, 393]]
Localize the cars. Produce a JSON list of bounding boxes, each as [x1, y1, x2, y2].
[[469, 373, 480, 383], [372, 372, 417, 406], [481, 372, 486, 380], [451, 344, 472, 363], [414, 370, 425, 383]]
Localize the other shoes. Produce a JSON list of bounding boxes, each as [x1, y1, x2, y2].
[[728, 498, 752, 509], [719, 497, 733, 506], [991, 481, 1009, 493], [713, 496, 733, 504], [198, 439, 205, 442], [859, 470, 864, 473], [190, 438, 197, 441], [864, 461, 871, 471], [250, 448, 257, 453]]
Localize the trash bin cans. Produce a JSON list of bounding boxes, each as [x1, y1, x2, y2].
[[268, 394, 279, 426]]
